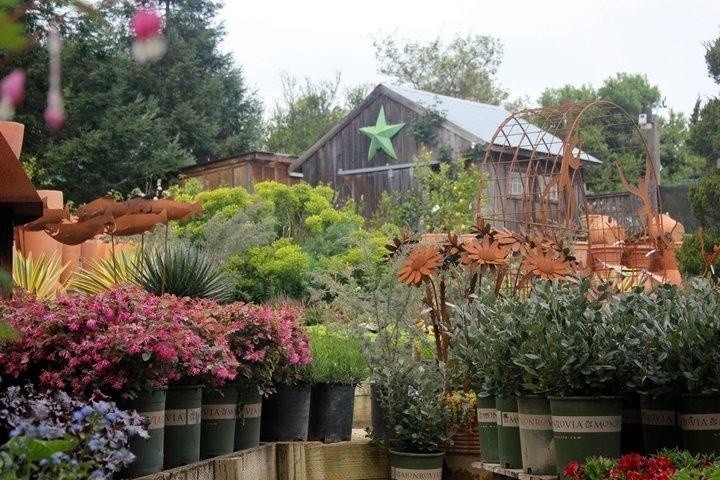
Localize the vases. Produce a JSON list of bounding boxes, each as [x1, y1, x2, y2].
[[566, 212, 686, 272]]
